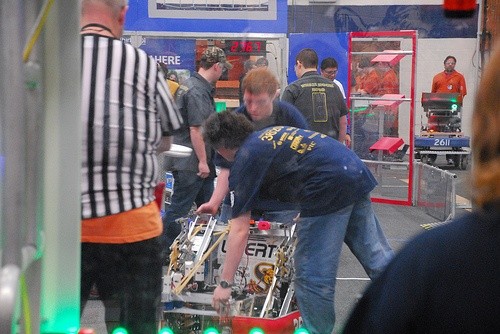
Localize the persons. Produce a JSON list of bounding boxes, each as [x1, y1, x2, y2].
[[76, 1, 500, 334]]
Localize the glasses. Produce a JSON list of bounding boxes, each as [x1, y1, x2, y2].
[[446, 62, 455, 65], [323, 71, 338, 74]]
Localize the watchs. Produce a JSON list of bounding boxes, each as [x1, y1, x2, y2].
[[219, 279, 234, 288]]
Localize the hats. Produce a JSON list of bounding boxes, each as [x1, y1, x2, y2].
[[200, 47, 233, 69]]
